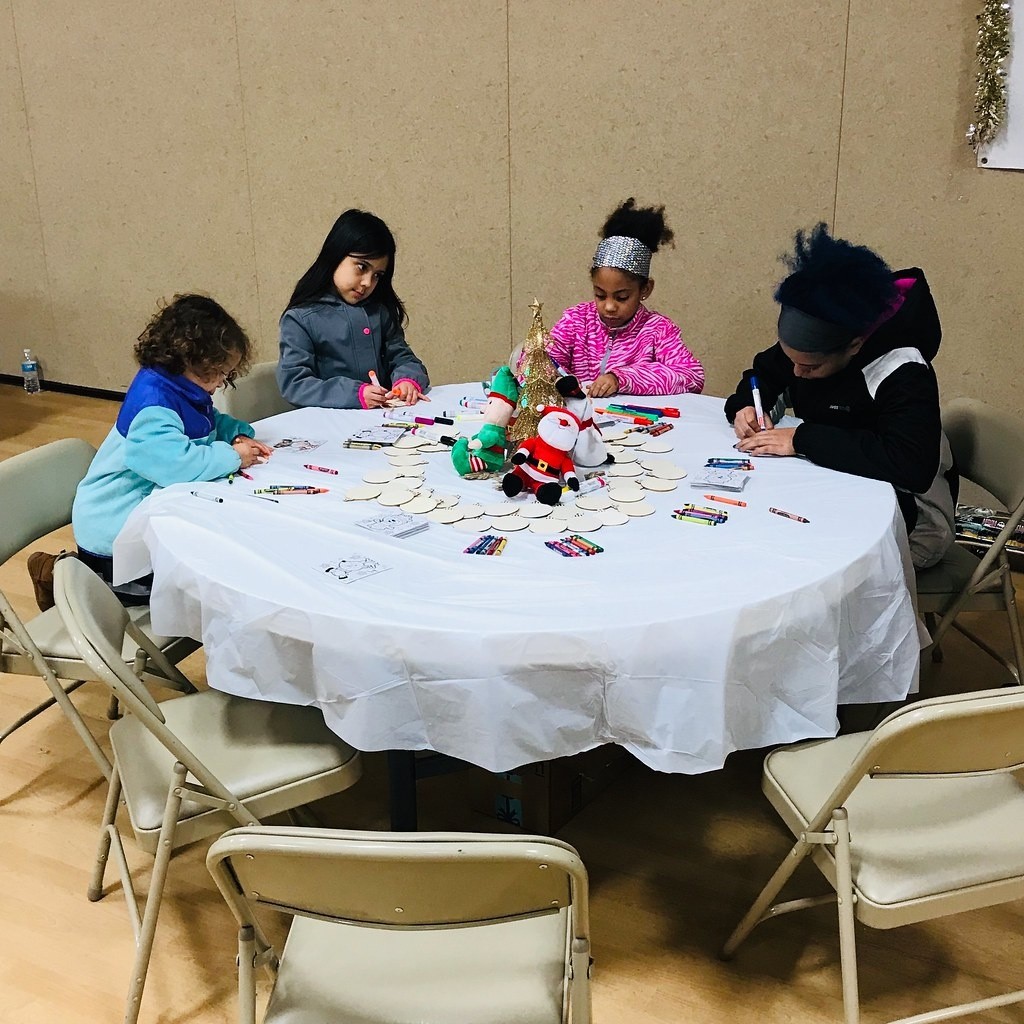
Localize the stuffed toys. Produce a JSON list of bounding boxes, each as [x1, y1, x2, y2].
[[450, 367, 518, 475], [502, 404, 579, 506], [556, 376, 615, 467]]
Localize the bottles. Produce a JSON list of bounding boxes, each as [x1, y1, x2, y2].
[[21, 349, 40, 395]]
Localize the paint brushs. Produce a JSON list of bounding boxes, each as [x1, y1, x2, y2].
[[624, 422, 674, 437], [545, 534, 605, 557], [229, 437, 330, 495], [342, 443, 381, 450], [443, 410, 484, 417], [368, 371, 379, 386], [304, 465, 338, 475], [460, 396, 488, 408], [559, 474, 607, 502], [550, 357, 568, 377], [404, 411, 454, 426], [671, 457, 755, 527], [769, 507, 810, 523], [594, 403, 681, 424], [751, 377, 765, 432], [249, 496, 279, 503], [457, 415, 486, 421], [383, 412, 434, 425], [191, 491, 224, 503], [463, 535, 507, 556]]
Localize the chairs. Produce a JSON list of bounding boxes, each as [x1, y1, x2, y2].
[[914, 399, 1024, 688], [721, 685, 1024, 1024], [52, 557, 363, 1024], [0, 438, 203, 786], [210, 361, 300, 427], [205, 825, 594, 1024]]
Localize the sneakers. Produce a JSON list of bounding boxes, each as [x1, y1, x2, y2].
[[27, 548, 67, 613]]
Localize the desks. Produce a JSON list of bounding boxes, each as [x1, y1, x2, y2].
[[111, 381, 932, 837]]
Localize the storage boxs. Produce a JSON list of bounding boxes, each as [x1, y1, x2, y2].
[[953, 502, 1024, 575]]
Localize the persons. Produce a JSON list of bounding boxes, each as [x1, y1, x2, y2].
[[517, 197, 705, 396], [725, 222, 942, 570], [273, 209, 432, 406], [26, 293, 276, 612]]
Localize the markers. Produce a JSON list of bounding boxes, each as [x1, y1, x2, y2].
[[411, 428, 457, 446]]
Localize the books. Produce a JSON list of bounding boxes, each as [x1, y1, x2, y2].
[[955, 503, 1024, 554]]
